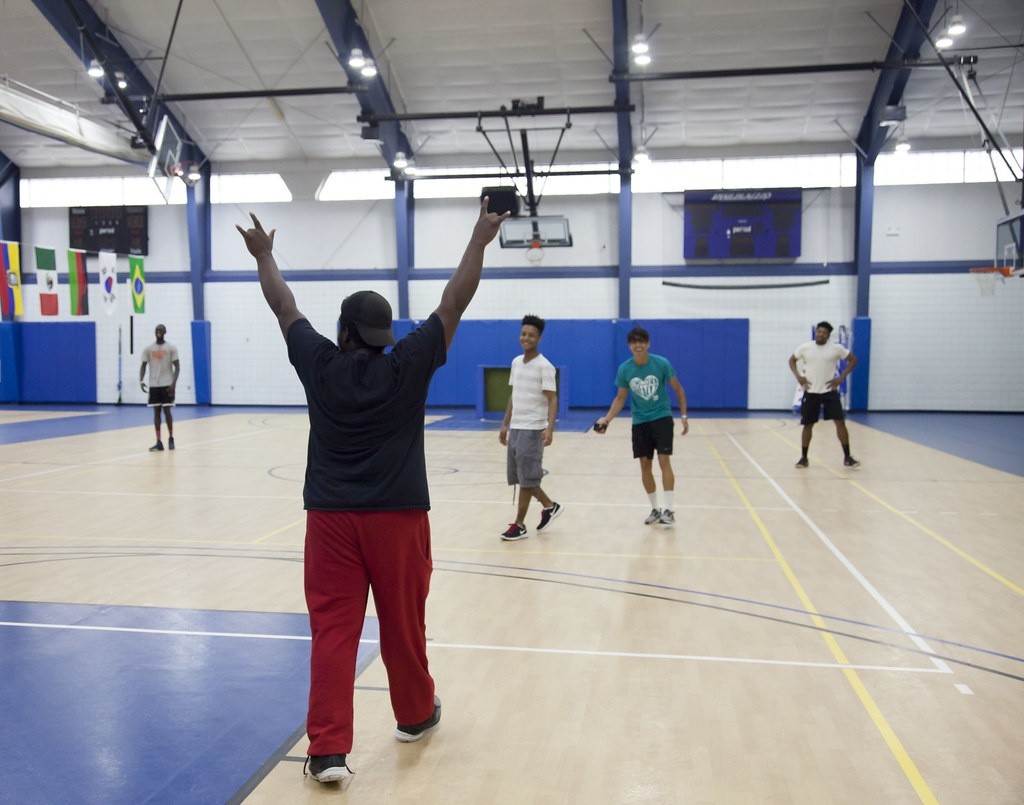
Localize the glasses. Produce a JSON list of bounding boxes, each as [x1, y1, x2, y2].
[[630, 338, 644, 342]]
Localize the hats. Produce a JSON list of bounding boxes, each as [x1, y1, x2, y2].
[[627, 328, 648, 342], [341, 291, 395, 346]]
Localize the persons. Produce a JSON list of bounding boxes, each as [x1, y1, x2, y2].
[[593, 327, 688, 524], [235, 195, 511, 783], [499, 315, 565, 540], [790, 322, 860, 468], [140, 324, 180, 451]]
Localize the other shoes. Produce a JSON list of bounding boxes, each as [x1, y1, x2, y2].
[[169, 437, 174, 449], [843, 456, 860, 467], [645, 508, 661, 524], [659, 510, 675, 523], [150, 442, 164, 451], [796, 457, 808, 467]]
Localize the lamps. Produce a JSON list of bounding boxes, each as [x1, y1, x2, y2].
[[392, 150, 409, 168], [935, 1, 954, 48], [632, 32, 653, 66], [404, 157, 416, 175], [86, 53, 105, 76], [893, 96, 911, 160], [947, 0, 967, 36], [349, 46, 364, 66], [361, 56, 378, 78], [114, 72, 128, 88]]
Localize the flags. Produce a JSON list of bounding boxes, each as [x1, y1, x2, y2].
[[68, 248, 88, 315], [128, 254, 145, 313], [0, 241, 22, 316], [97, 251, 118, 305], [35, 247, 58, 315]]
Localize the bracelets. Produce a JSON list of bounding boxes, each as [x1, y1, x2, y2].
[[140, 381, 143, 384], [681, 415, 687, 419]]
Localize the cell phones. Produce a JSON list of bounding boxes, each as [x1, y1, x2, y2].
[[593, 424, 607, 432]]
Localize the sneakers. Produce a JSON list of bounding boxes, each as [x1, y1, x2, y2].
[[500, 523, 528, 540], [536, 502, 565, 530], [303, 753, 355, 782], [394, 695, 441, 742]]
